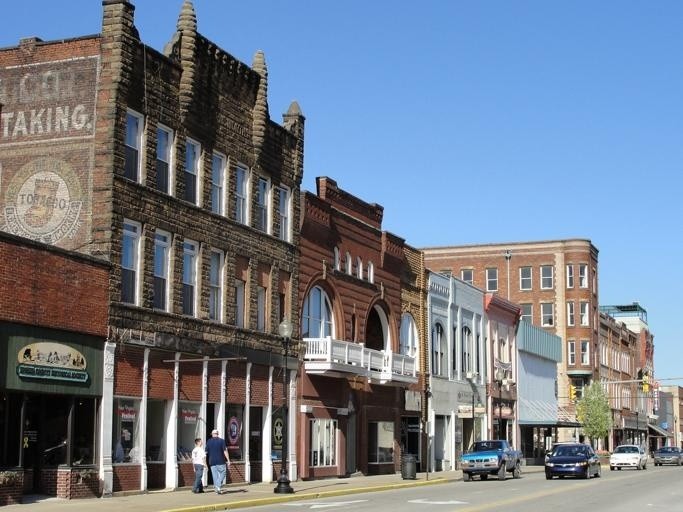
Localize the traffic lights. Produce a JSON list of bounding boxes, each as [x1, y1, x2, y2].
[[570, 385, 576, 400]]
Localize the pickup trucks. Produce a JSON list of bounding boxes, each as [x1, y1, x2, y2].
[[458, 439, 523, 482]]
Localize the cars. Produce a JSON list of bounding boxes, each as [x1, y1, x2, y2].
[[608, 444, 647, 471], [544, 442, 582, 464], [543, 446, 601, 479], [651, 446, 683, 466]]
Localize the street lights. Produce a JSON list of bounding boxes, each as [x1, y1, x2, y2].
[[635, 408, 639, 446], [495, 368, 504, 441], [273, 316, 294, 495], [674, 415, 677, 447]]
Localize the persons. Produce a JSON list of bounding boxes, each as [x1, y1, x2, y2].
[[190, 437, 206, 492], [205, 428, 230, 494]]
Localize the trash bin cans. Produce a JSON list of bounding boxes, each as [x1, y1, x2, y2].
[[401, 452, 417, 480]]
